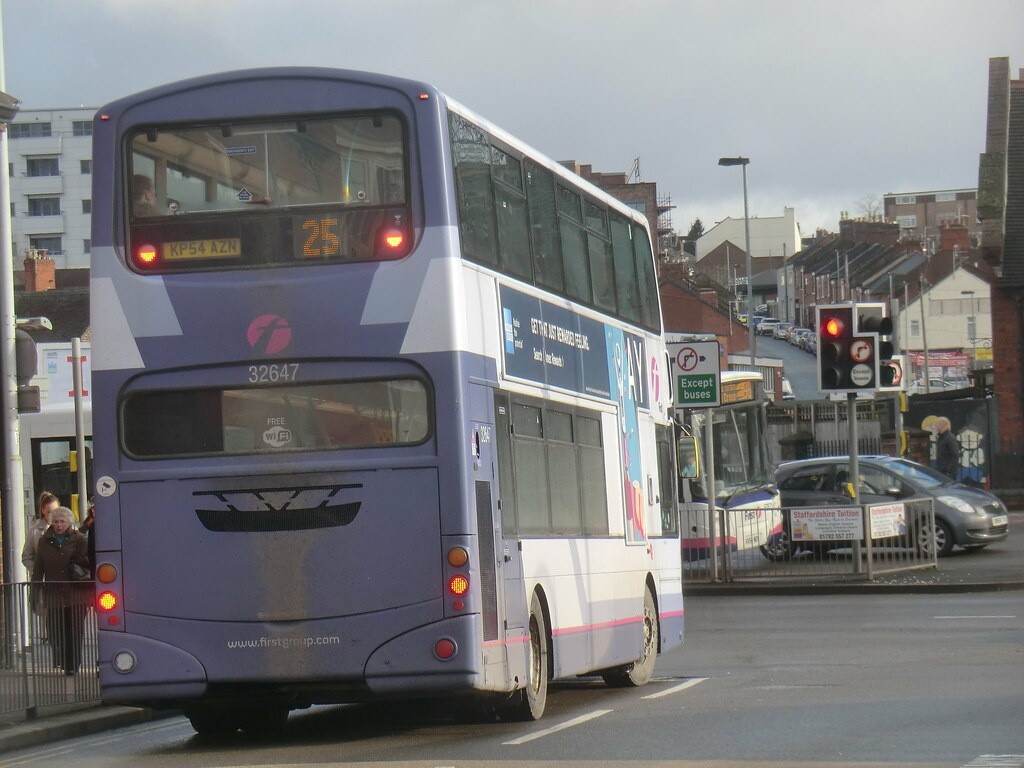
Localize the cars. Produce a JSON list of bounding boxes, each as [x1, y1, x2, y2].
[[738, 315, 816, 355], [907, 378, 954, 395]]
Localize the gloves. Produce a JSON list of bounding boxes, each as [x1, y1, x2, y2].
[[29, 595, 40, 612]]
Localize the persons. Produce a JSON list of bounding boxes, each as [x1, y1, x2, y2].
[[836, 471, 874, 493], [22, 491, 78, 668], [936, 417, 958, 480], [30, 507, 90, 675], [78, 496, 99, 667], [133, 173, 163, 216], [681, 449, 696, 477]]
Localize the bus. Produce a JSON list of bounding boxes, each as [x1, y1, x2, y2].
[[94, 67, 701, 739], [16, 403, 94, 597], [675, 371, 784, 561]]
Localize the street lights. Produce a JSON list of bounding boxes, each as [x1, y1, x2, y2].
[[732, 264, 741, 298], [962, 290, 976, 372], [718, 156, 756, 371]]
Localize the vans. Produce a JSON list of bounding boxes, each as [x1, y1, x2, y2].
[[760, 454, 1009, 563]]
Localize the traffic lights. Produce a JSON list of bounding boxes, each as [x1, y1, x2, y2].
[[815, 304, 879, 392], [852, 302, 893, 389]]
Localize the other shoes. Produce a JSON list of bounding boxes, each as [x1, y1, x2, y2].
[[64, 667, 80, 676]]
